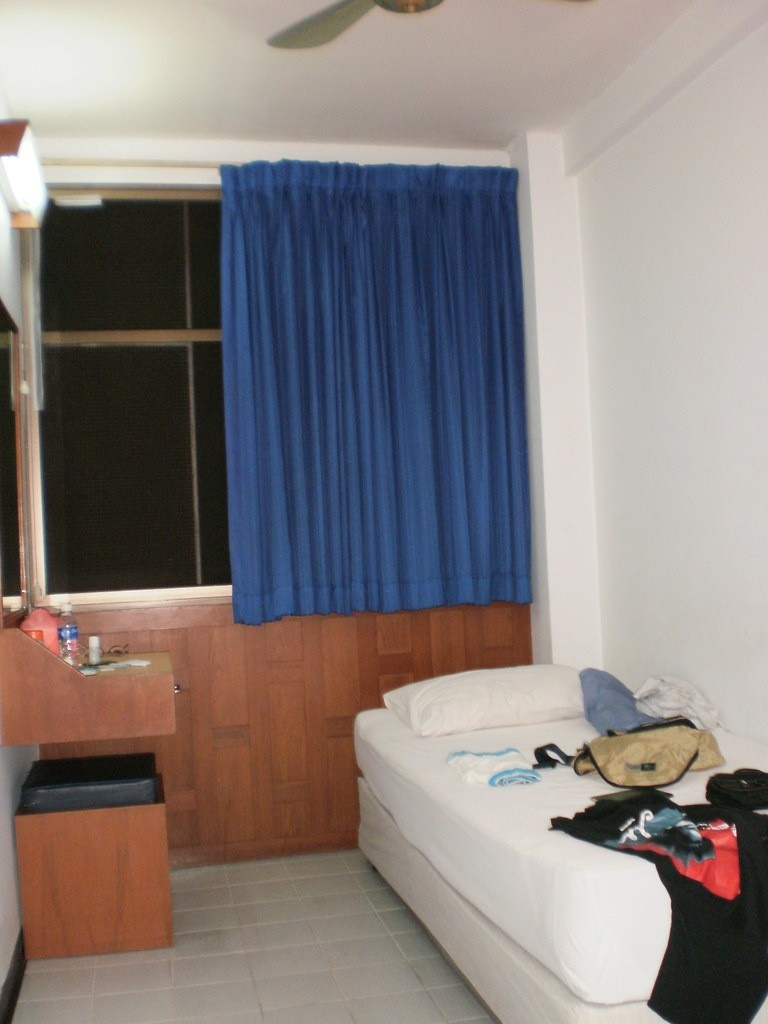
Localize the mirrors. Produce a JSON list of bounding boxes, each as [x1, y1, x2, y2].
[[0, 298, 29, 629]]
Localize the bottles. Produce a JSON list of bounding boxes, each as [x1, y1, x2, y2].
[[58, 602, 79, 669], [88, 636, 100, 664]]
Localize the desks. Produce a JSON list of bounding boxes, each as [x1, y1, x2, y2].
[[1, 629, 172, 744]]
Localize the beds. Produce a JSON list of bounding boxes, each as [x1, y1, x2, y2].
[[354, 696, 768, 1023]]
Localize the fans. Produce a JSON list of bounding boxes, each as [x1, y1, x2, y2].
[[264, 0, 608, 50]]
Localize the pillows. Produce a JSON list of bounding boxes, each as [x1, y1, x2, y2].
[[380, 661, 593, 741]]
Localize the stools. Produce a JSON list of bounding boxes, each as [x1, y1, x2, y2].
[[12, 752, 177, 959]]
